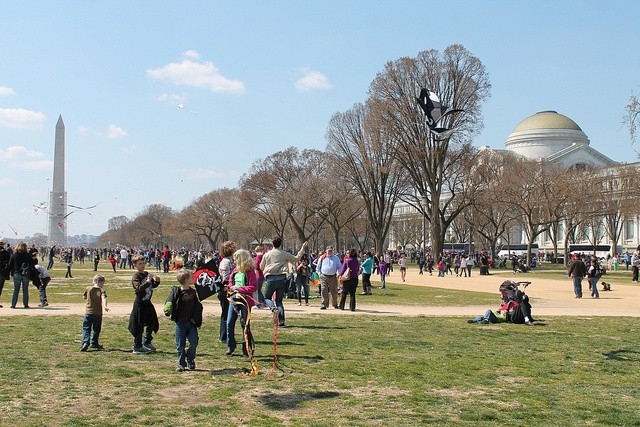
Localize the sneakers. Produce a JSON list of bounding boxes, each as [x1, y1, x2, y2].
[[38, 303, 44, 307], [273, 309, 279, 324], [279, 323, 285, 328], [176, 364, 185, 372], [226, 345, 236, 355], [43, 302, 48, 306], [91, 344, 104, 349], [298, 303, 301, 306], [187, 358, 195, 369], [133, 348, 152, 354], [321, 307, 326, 309], [80, 343, 88, 351], [351, 307, 355, 311], [339, 306, 344, 310], [305, 303, 309, 306], [143, 343, 156, 351], [335, 306, 338, 309]]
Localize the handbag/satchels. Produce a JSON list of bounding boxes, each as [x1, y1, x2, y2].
[[20, 262, 30, 275], [340, 259, 352, 281]]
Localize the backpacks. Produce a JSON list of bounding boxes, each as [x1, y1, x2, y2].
[[171, 287, 198, 324]]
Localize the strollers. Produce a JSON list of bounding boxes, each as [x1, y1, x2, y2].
[[500, 280, 531, 314]]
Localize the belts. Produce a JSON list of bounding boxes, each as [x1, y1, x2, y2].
[[323, 274, 336, 277]]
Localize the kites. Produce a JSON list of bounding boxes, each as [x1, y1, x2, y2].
[[178, 103, 198, 117], [411, 88, 465, 141], [267, 292, 279, 382], [309, 272, 320, 287], [32, 201, 101, 219], [8, 224, 17, 236], [192, 258, 259, 375]]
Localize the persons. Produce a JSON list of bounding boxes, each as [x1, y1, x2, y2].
[[408, 247, 556, 280], [80, 274, 111, 351], [601, 282, 610, 291], [253, 246, 265, 309], [32, 258, 51, 308], [346, 249, 350, 257], [8, 242, 32, 308], [285, 250, 296, 277], [568, 254, 586, 298], [467, 309, 508, 324], [58, 223, 65, 228], [133, 247, 154, 267], [384, 251, 397, 275], [0, 242, 10, 308], [398, 254, 406, 282], [217, 240, 237, 340], [316, 246, 342, 309], [360, 251, 373, 295], [163, 269, 203, 371], [128, 254, 160, 353], [604, 246, 640, 283], [156, 244, 218, 273], [41, 244, 132, 278], [334, 251, 349, 264], [508, 301, 534, 326], [260, 237, 309, 326], [338, 249, 359, 311], [588, 260, 602, 297], [29, 244, 38, 258], [296, 254, 311, 306], [372, 251, 380, 274], [589, 255, 597, 290], [224, 249, 258, 357], [5, 243, 13, 255], [311, 251, 323, 296], [378, 254, 387, 289]]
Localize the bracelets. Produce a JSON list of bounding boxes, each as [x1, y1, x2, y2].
[[235, 286, 238, 290]]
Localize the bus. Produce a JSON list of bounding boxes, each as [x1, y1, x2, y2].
[[442, 242, 475, 256], [569, 244, 613, 258], [495, 243, 538, 259]]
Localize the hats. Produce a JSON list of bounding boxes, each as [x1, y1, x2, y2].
[[0, 238, 6, 243], [326, 246, 333, 251]]
